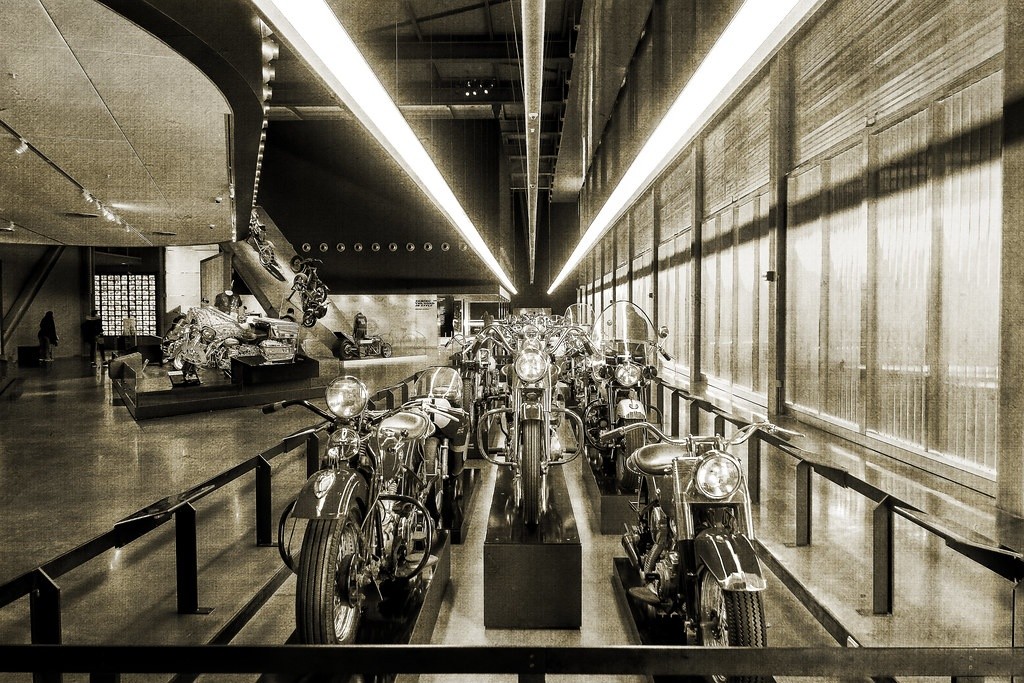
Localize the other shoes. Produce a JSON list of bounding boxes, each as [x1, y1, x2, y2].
[[40, 358, 54, 362]]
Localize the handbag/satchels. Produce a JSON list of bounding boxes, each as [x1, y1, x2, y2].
[[98, 338, 104, 344]]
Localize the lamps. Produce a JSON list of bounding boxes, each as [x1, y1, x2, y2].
[[16, 139, 28, 155]]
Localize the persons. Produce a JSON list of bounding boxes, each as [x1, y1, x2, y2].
[[626, 304, 649, 365], [84, 310, 110, 368], [37, 311, 59, 362], [481, 311, 495, 334]]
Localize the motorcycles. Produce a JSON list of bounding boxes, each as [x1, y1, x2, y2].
[[340, 333, 392, 359], [600, 421, 806, 683], [290, 255, 328, 327], [161, 312, 258, 379], [260, 376, 470, 645], [247, 209, 275, 266], [405, 300, 672, 518], [463, 323, 595, 528]]
